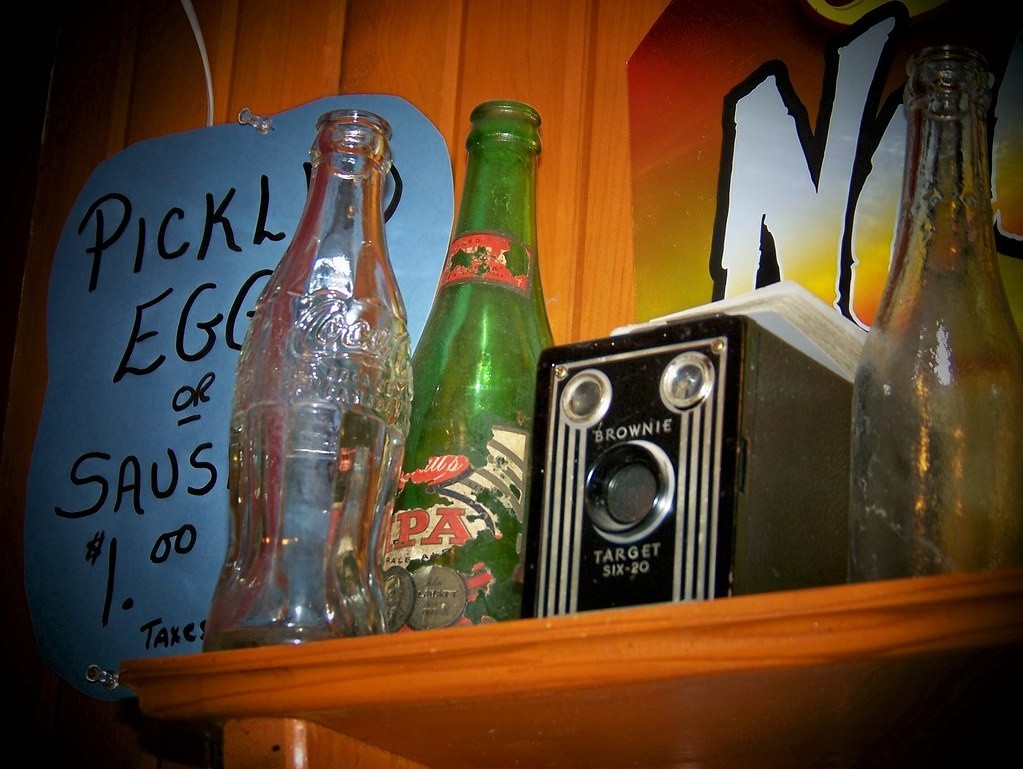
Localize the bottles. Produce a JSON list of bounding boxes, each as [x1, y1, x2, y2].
[[380, 100, 554, 631], [849, 45, 1023, 584], [202, 106, 415, 651]]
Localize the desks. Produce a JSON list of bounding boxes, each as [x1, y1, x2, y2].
[[116, 566, 1023, 769]]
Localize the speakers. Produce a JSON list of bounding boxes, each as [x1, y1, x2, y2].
[[518, 317, 856, 619]]
